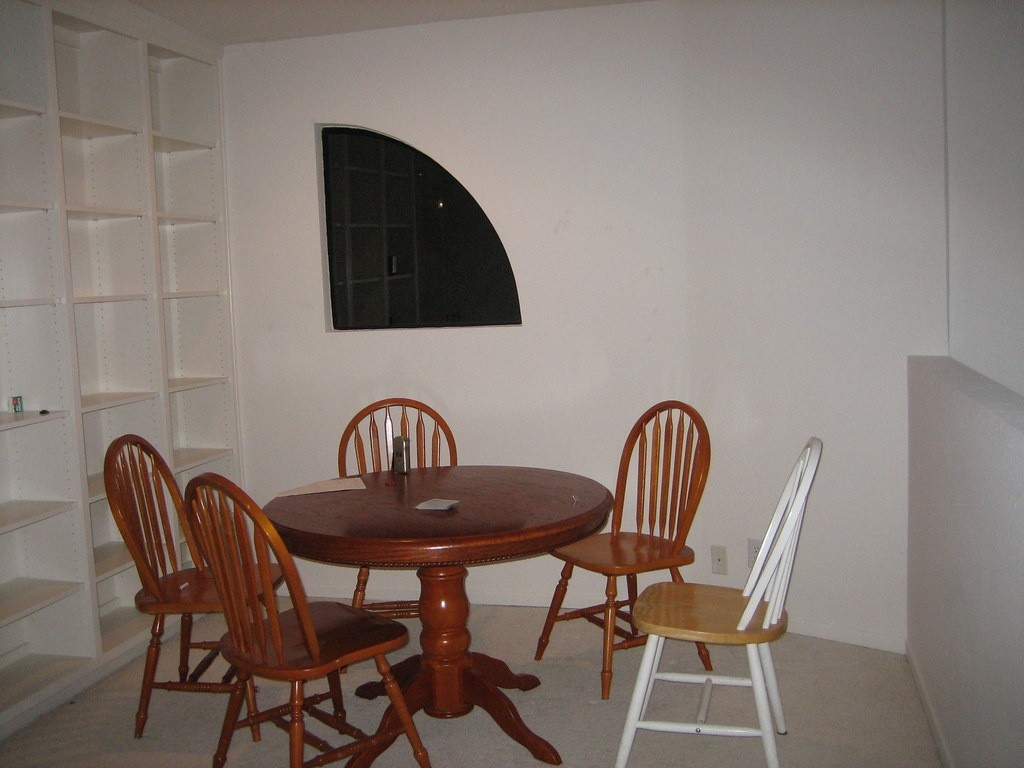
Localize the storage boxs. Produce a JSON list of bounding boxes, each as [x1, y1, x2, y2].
[[8, 396, 23, 413]]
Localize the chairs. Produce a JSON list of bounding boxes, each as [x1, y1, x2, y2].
[[185, 471, 432, 768], [101, 435, 287, 744], [535, 400, 715, 700], [607, 436, 824, 768], [336, 397, 459, 677]]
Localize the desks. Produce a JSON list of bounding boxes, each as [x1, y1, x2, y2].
[[262, 465, 617, 768]]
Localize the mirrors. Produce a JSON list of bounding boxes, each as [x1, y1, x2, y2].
[[312, 122, 523, 332]]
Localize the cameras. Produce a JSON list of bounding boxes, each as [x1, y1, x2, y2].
[[393, 436, 410, 473]]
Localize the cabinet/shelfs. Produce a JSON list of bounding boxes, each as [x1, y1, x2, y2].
[[0, 0, 251, 746], [327, 134, 421, 328]]
